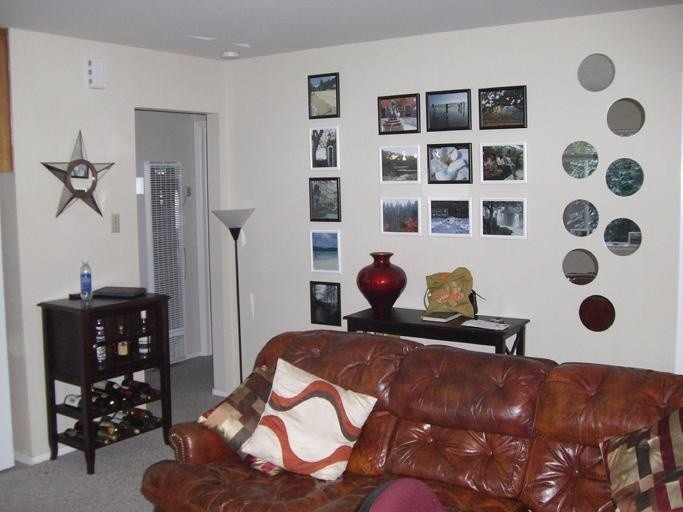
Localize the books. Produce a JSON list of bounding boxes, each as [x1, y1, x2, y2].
[[421, 312, 463, 323], [93, 286, 147, 299]]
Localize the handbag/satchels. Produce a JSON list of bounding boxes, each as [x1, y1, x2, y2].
[[424, 267, 475, 318]]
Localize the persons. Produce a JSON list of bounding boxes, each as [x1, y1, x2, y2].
[[484, 94, 521, 113], [485, 149, 524, 180]]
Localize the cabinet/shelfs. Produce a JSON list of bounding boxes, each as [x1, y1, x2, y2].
[[37, 292, 173, 474]]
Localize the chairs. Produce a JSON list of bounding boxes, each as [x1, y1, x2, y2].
[[358, 477, 447, 512]]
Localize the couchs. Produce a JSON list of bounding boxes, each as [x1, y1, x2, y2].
[[140, 330, 683, 512]]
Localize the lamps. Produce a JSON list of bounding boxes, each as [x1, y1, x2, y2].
[[213, 208, 255, 385]]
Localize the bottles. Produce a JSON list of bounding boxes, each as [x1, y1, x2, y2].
[[80, 259, 92, 306]]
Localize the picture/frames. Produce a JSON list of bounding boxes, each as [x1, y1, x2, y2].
[[307, 72, 341, 327], [72, 164, 89, 180], [376, 85, 526, 238]]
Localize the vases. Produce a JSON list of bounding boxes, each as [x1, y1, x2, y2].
[[357, 252, 406, 319]]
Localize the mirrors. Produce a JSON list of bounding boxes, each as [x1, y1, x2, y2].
[[562, 54, 645, 331], [41, 130, 115, 218]]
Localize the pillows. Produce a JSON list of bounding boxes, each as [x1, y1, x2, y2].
[[199, 365, 282, 478], [242, 356, 378, 481], [598, 411, 683, 512]]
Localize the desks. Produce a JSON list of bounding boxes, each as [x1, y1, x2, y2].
[[343, 308, 530, 356]]
